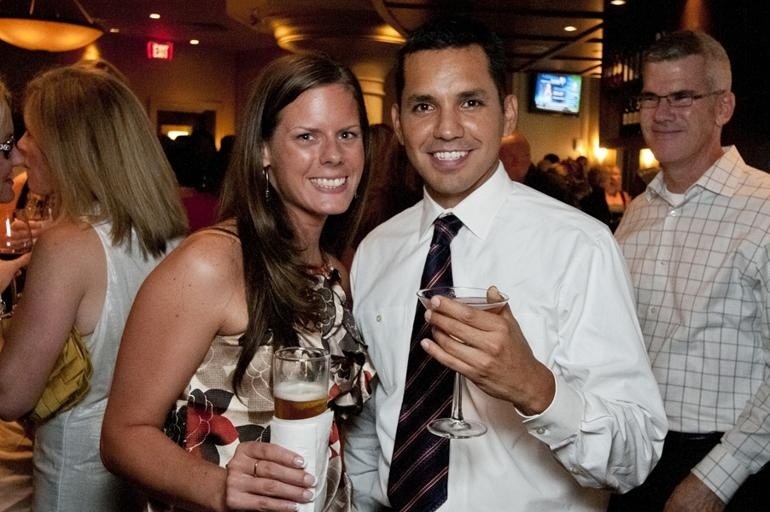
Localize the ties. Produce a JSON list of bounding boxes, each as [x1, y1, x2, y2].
[[387, 217, 463, 509]]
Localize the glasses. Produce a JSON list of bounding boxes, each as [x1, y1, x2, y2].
[[2, 136, 15, 158], [633, 89, 723, 109]]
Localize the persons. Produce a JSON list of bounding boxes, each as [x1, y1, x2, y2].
[[1, 107, 644, 267], [340, 21, 670, 510], [0, 80, 50, 511], [0, 62, 191, 510], [95, 49, 383, 511], [600, 27, 770, 510]]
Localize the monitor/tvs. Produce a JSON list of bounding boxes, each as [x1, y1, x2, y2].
[[526, 67, 583, 117]]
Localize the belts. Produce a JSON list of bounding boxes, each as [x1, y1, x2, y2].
[[664, 431, 724, 448]]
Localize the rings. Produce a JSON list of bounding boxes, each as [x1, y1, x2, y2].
[[253, 457, 262, 475]]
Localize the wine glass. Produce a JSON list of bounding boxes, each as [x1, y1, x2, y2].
[[415, 286, 511, 441], [0, 208, 35, 318]]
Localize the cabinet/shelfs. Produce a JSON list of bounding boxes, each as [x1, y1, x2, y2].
[[598, 0, 710, 150]]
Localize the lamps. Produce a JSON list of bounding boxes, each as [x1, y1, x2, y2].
[[0, 0, 105, 54]]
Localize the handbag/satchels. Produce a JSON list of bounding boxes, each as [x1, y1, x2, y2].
[[1, 306, 92, 433]]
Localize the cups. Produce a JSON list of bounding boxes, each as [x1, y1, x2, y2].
[[272, 345, 333, 420]]
